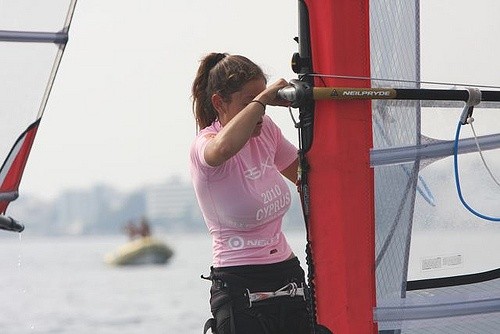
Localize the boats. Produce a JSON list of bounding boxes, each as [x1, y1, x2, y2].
[[107, 235, 173, 264]]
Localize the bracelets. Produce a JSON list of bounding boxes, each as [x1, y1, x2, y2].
[[249, 100, 265, 110]]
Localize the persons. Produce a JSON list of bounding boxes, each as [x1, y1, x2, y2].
[[188, 53, 332, 334]]
